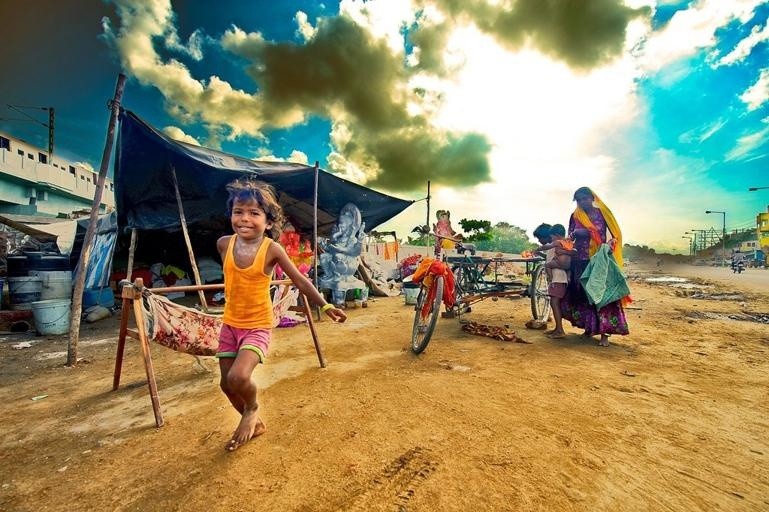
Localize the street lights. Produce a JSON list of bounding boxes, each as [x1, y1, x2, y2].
[[705, 211, 726, 267], [691, 229, 703, 250], [749, 187, 769, 191], [685, 232, 696, 255], [681, 236, 692, 256]]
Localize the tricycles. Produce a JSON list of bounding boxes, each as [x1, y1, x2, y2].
[[407, 225, 553, 355]]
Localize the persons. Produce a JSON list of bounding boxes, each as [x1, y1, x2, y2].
[[216, 179, 346, 451], [534, 224, 571, 338], [730, 250, 746, 269], [560, 187, 632, 346], [537, 224, 573, 284]]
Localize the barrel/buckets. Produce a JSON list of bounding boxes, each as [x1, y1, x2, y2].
[[30, 297, 70, 336], [7, 257, 28, 277], [403, 281, 426, 306], [28, 271, 73, 300], [6, 275, 42, 311]]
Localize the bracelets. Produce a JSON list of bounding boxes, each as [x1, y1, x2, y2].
[[320, 303, 334, 313]]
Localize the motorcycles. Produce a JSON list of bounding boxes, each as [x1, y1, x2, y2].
[[730, 257, 745, 274]]
